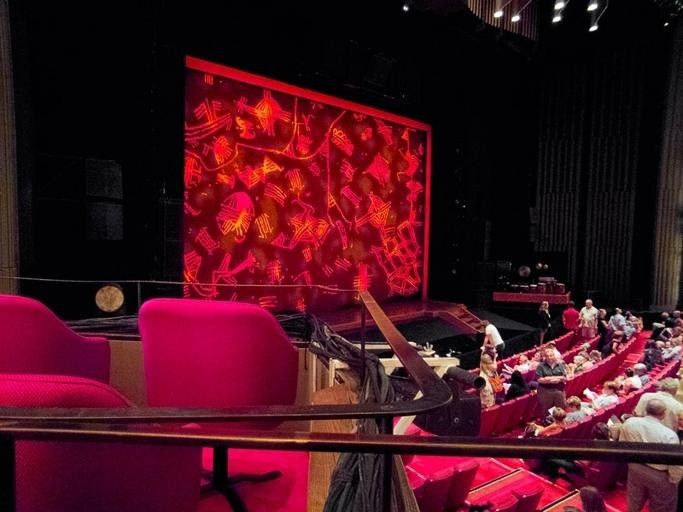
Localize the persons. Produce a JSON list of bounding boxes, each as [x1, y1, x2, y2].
[[634, 311, 682, 386], [518, 363, 682, 512], [563, 300, 643, 359], [537, 301, 551, 346], [480, 320, 506, 408], [506, 343, 603, 418]]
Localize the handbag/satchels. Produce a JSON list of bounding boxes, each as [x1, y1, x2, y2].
[[489, 375, 503, 393]]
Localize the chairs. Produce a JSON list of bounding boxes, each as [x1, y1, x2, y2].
[[0, 293, 683, 512]]
